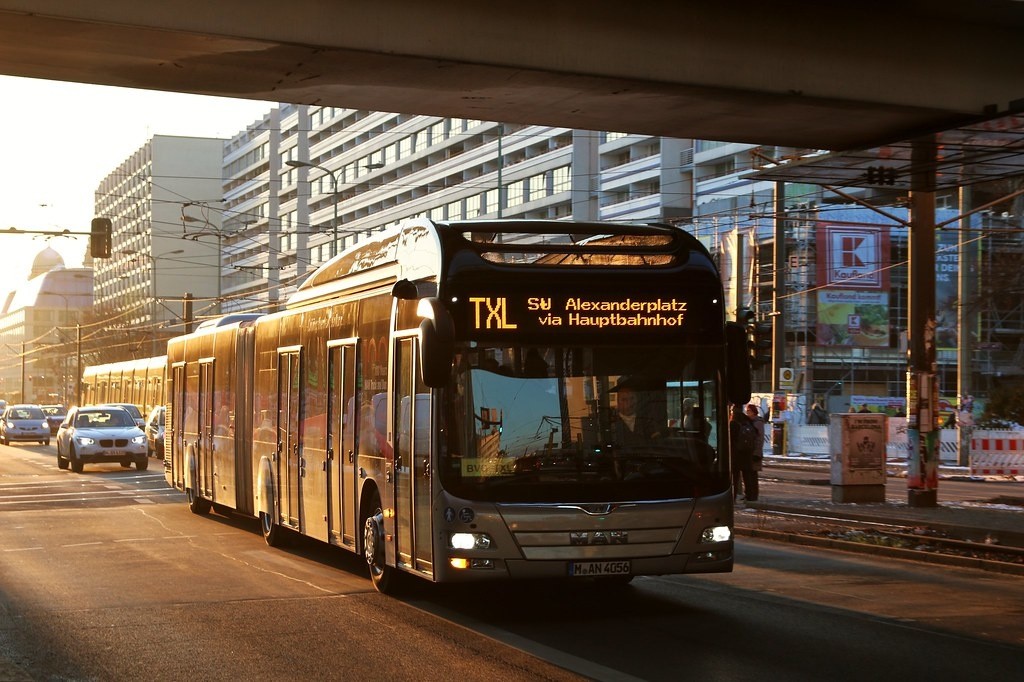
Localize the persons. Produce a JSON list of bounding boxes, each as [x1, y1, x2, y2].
[[849, 403, 889, 413], [943, 402, 974, 441], [682, 398, 713, 444], [606, 375, 667, 481], [893, 406, 906, 417], [807, 403, 830, 425], [730, 403, 765, 502]]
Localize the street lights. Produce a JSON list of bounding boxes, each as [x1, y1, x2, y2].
[[120, 248, 184, 357], [181, 215, 257, 314], [42, 292, 69, 405], [74, 274, 130, 364], [286, 160, 386, 258]]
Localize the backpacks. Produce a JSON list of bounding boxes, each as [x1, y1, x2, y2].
[[732, 418, 755, 451]]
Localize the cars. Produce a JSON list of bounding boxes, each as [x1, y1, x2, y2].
[[102, 403, 148, 433], [57, 406, 149, 473], [40, 406, 69, 433], [145, 405, 166, 459], [0, 405, 51, 446]]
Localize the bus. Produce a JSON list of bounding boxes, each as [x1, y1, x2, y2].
[[162, 215, 755, 597], [83, 354, 167, 422]]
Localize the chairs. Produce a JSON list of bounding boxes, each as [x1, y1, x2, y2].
[[79, 415, 90, 426]]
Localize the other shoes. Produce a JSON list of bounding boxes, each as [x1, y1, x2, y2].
[[736, 496, 747, 504]]
